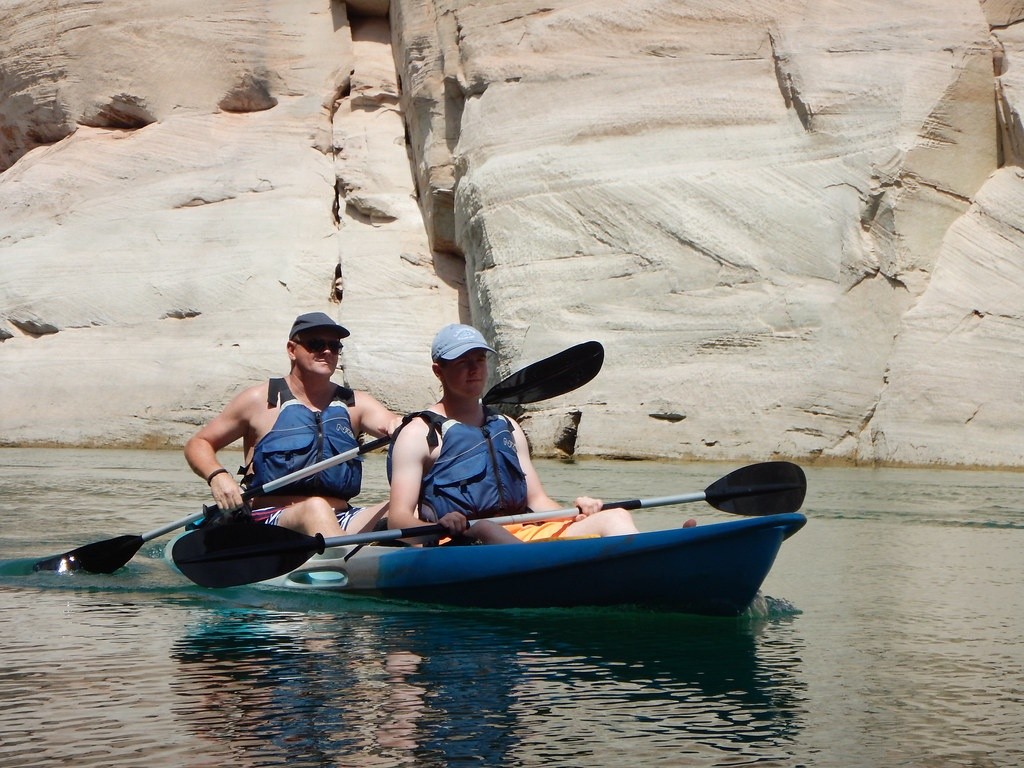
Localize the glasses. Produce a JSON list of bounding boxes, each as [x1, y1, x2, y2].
[[293, 338, 344, 355]]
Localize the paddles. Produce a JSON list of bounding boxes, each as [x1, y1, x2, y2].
[[31, 341, 605, 576], [171, 459, 807, 590]]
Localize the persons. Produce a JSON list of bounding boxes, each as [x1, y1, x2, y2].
[[387, 324, 696, 548], [185, 313, 404, 548]]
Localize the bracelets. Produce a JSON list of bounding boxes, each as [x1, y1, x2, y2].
[[207, 469, 228, 487]]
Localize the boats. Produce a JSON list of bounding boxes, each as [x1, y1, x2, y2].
[[163, 512, 807, 618]]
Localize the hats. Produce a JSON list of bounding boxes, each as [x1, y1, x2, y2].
[[289, 312, 350, 343], [431, 323, 498, 362]]
